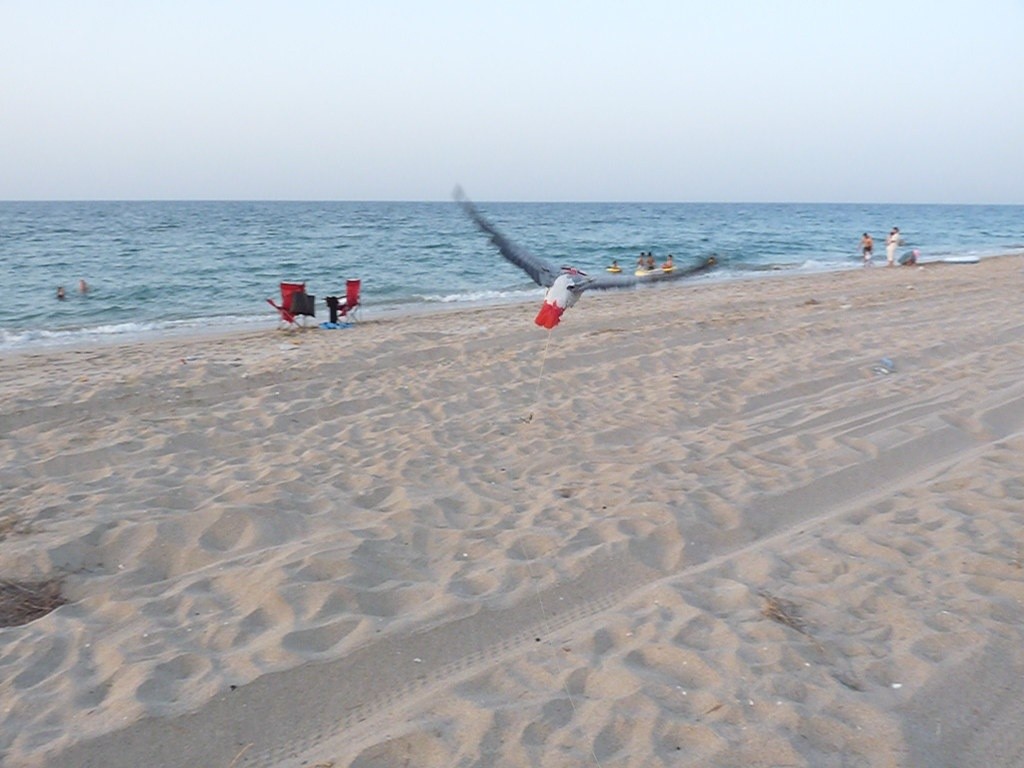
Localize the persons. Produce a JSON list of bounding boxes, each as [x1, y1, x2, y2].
[[57, 287, 65, 298], [899, 249, 920, 265], [664, 255, 672, 268], [612, 261, 617, 268], [709, 257, 716, 264], [857, 233, 872, 267], [639, 252, 653, 268], [886, 227, 899, 265], [79, 281, 86, 292]]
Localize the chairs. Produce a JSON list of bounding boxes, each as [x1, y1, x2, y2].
[[266, 281, 305, 330], [320, 279, 362, 329]]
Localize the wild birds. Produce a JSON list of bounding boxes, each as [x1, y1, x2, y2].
[[452, 181, 719, 330]]
